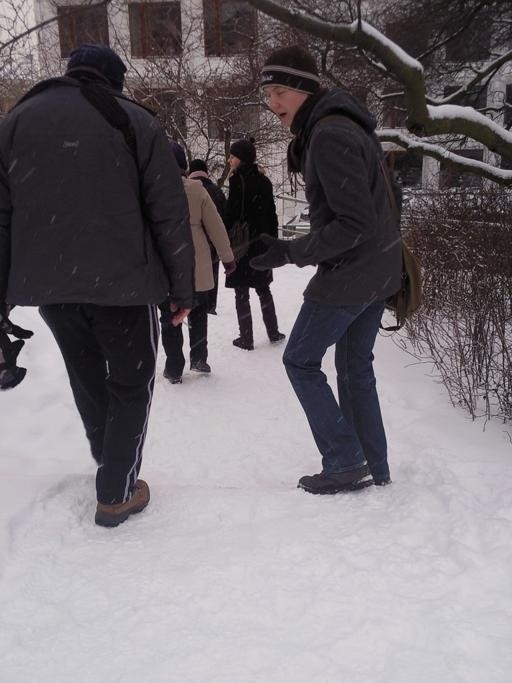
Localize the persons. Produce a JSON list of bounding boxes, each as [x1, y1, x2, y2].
[[225, 137, 286, 350], [248, 44, 405, 493], [0, 42, 195, 529], [187, 158, 225, 317], [160, 136, 236, 383]]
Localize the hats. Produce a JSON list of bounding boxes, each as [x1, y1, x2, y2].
[[66, 43, 127, 92], [229, 136, 256, 162], [189, 158, 208, 173], [260, 44, 320, 96], [169, 139, 188, 170]]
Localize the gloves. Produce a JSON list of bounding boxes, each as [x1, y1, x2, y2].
[[249, 232, 291, 271], [223, 260, 237, 274], [1, 314, 34, 390]]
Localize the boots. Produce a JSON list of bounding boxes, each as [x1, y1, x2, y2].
[[263, 315, 286, 343], [232, 320, 255, 350]]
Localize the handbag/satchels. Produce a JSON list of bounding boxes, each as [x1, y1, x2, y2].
[[227, 220, 255, 258], [382, 243, 424, 317]]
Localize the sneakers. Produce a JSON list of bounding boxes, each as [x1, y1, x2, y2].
[[373, 471, 393, 486], [163, 369, 183, 384], [296, 463, 375, 496], [190, 360, 211, 372], [94, 478, 151, 527]]
